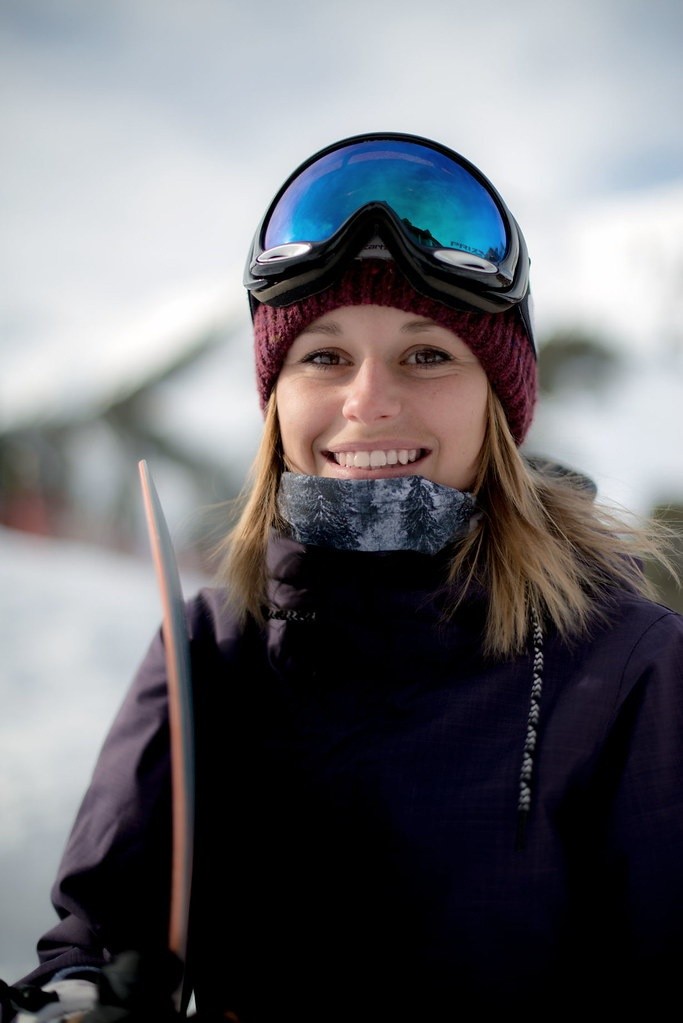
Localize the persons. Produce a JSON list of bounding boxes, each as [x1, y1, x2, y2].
[[12, 132, 683, 1023]]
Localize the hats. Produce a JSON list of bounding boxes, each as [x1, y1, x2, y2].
[[253, 247, 539, 452]]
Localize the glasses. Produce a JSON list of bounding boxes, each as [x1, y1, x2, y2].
[[244, 133, 539, 357]]
